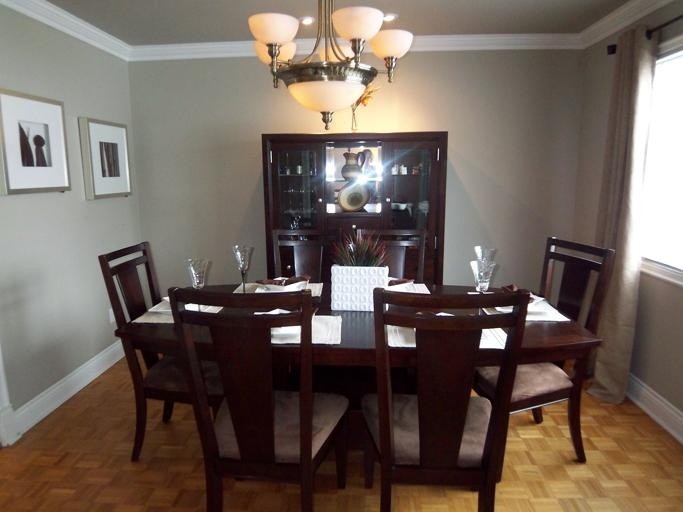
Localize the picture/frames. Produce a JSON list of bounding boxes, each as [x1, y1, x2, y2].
[[0, 88, 71, 196], [78, 116, 133, 200]]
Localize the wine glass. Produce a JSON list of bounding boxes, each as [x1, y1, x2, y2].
[[185, 257, 209, 312], [470, 246, 498, 315], [231, 240, 254, 293]]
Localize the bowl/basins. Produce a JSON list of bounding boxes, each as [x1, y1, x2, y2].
[[392, 201, 429, 212]]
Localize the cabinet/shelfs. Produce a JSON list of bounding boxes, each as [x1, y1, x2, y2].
[[262, 130, 448, 286]]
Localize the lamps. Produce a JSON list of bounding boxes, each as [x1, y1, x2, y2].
[[248, 0, 414, 130]]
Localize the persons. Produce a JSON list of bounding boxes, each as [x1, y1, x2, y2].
[[359, 149, 377, 204]]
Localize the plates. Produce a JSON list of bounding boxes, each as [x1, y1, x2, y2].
[[494, 291, 545, 312], [255, 279, 309, 294], [337, 183, 369, 212], [148, 299, 212, 314]]
